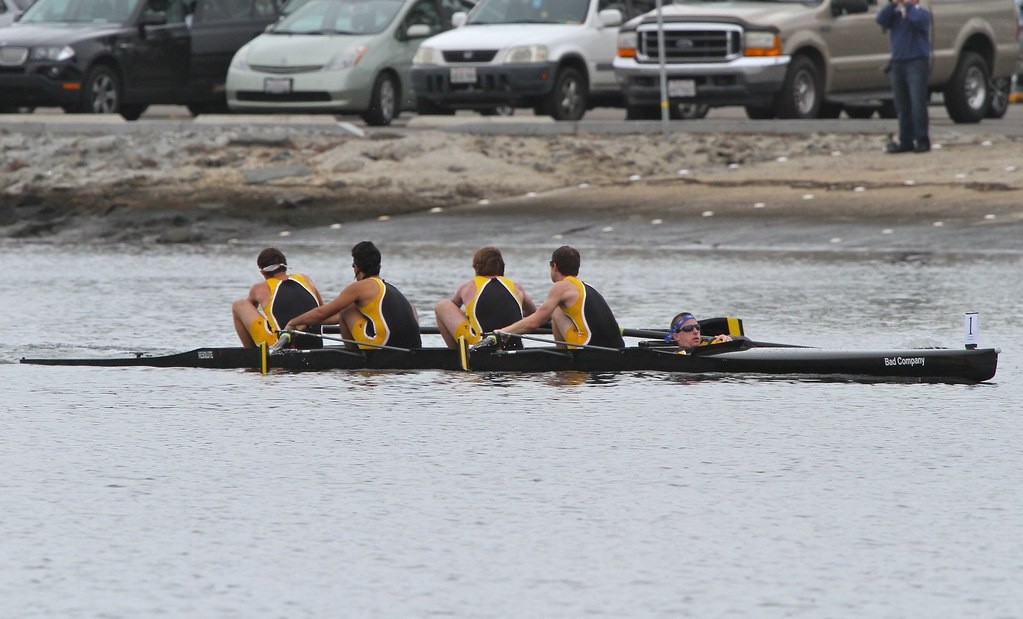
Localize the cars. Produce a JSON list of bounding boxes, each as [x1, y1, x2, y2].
[[225, 0, 516, 125], [1, 0, 280, 122], [0, 0, 45, 24]]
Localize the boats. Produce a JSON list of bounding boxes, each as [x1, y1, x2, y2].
[[19, 343, 1000, 384]]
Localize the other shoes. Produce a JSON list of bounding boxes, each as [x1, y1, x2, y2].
[[891, 140, 914, 152], [914, 138, 931, 151]]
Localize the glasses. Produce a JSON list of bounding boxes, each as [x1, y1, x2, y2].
[[677, 324, 700, 333], [550, 261, 554, 266]]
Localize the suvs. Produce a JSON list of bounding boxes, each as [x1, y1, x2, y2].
[[613, 1, 1023, 124], [409, 0, 712, 121]]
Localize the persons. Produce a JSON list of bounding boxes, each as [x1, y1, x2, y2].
[[232, 247, 324, 349], [666, 313, 733, 356], [494, 246, 625, 349], [285, 241, 422, 348], [875, 0, 932, 153], [435, 247, 537, 349]]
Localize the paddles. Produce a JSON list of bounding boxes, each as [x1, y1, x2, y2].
[[519, 316, 745, 336], [541, 321, 670, 339], [255, 332, 291, 374], [321, 325, 440, 335]]
